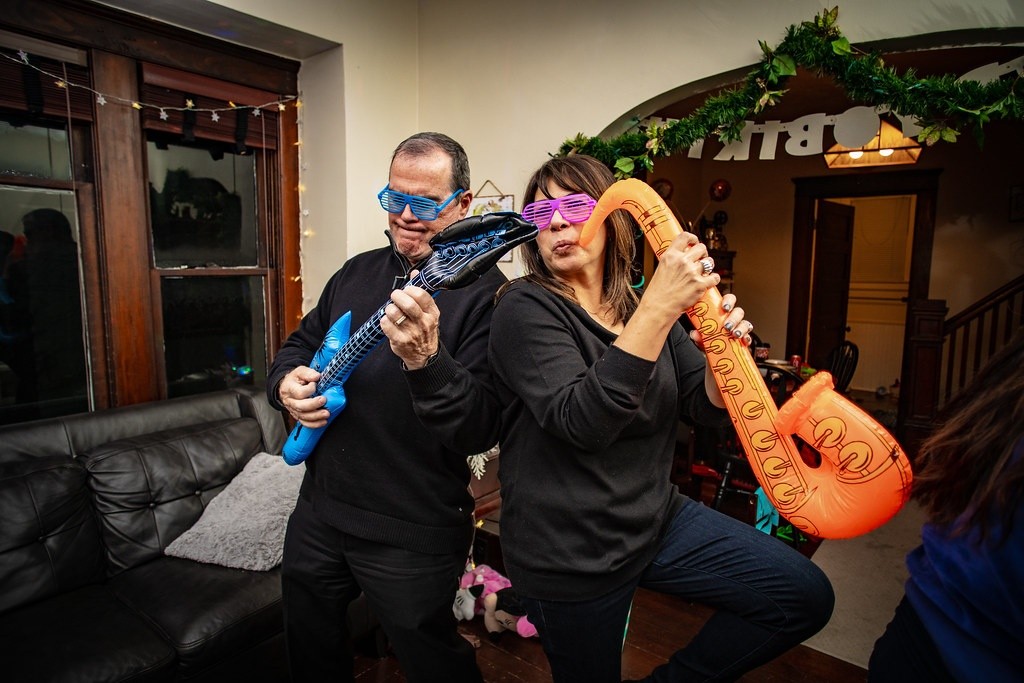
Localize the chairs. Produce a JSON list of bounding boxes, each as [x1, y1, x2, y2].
[[747, 330, 770, 359], [712, 364, 806, 546], [820, 341, 860, 395]]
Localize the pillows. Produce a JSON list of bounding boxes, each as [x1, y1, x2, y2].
[[165, 453, 306, 573]]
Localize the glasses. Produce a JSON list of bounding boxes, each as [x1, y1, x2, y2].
[[377, 182, 464, 220], [521, 194, 596, 230]]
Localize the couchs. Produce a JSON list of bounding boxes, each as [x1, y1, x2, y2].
[[1, 382, 290, 683]]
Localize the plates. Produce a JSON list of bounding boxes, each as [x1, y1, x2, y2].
[[765, 359, 787, 365]]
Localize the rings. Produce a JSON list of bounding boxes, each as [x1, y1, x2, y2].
[[699, 260, 715, 276], [392, 315, 407, 326]]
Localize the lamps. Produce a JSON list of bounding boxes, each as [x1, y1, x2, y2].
[[823, 118, 922, 169]]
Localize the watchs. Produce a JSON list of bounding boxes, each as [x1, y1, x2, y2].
[[401, 349, 439, 372]]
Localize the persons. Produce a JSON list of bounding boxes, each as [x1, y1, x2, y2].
[[267, 132, 510, 682], [486, 154, 836, 683]]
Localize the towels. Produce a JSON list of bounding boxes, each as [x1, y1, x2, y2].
[[754, 486, 781, 535]]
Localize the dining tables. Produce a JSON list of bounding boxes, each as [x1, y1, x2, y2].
[[754, 358, 837, 400]]
